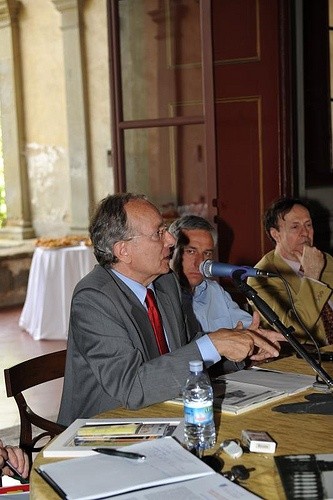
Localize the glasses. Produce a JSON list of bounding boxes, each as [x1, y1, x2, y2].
[[119, 224, 168, 240]]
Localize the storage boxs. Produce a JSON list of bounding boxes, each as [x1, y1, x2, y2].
[[241, 429, 276, 453]]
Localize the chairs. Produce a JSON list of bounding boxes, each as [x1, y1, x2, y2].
[[4, 348, 68, 484]]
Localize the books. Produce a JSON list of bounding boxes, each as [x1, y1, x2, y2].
[[165, 370, 323, 415], [35, 436, 267, 500]]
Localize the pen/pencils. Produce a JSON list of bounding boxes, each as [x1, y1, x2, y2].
[[91, 448, 147, 460]]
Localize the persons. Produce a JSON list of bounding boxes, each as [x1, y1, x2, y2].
[[0, 440, 29, 478], [57, 192, 287, 427], [168, 215, 253, 332], [247, 197, 333, 356]]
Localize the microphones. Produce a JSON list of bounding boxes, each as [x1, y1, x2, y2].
[[199, 259, 279, 278]]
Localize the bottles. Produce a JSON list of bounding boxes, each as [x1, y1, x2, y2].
[[183, 360, 215, 450]]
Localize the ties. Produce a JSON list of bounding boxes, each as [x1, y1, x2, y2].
[[145, 288, 170, 357]]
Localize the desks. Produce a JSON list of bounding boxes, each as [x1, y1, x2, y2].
[[19, 242, 100, 340], [30, 342, 333, 500]]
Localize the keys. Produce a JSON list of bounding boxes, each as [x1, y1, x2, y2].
[[232, 465, 256, 480]]
